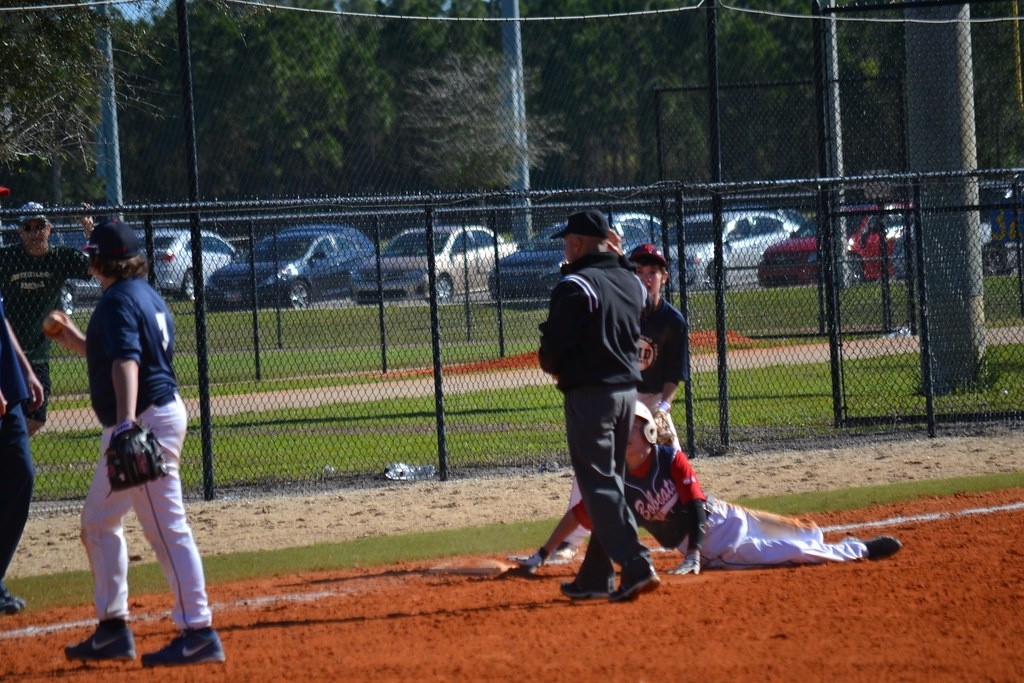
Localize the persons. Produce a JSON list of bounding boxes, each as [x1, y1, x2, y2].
[[550, 245, 691, 560], [0, 202, 94, 435], [0, 302, 43, 614], [538, 210, 662, 601], [47, 218, 226, 664], [517, 401, 903, 575]]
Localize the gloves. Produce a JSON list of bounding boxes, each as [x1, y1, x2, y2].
[[666, 554, 701, 575], [507, 552, 543, 575]]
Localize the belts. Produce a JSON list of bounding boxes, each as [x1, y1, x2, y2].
[[153, 393, 175, 408], [705, 494, 714, 520]]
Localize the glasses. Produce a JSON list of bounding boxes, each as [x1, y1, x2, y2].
[[23, 224, 46, 232]]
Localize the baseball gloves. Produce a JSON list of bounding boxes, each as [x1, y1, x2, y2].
[[101, 415, 170, 500], [653, 411, 676, 447]]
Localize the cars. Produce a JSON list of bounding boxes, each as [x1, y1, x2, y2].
[[74, 227, 248, 300], [626, 209, 804, 294], [889, 173, 1024, 280], [348, 224, 519, 304], [488, 211, 666, 301], [1, 220, 104, 299], [757, 201, 910, 288], [206, 224, 376, 313]]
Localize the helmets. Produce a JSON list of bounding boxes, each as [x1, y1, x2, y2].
[[633, 400, 658, 444], [628, 244, 668, 267]]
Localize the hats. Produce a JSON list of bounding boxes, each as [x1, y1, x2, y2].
[[550, 210, 610, 239], [82, 219, 141, 258], [19, 201, 47, 222]]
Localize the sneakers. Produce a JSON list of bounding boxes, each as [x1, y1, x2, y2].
[[0, 582, 26, 613], [863, 535, 901, 558], [606, 564, 662, 602], [555, 542, 578, 559], [561, 579, 612, 599], [64, 624, 136, 662], [140, 628, 225, 668]]
